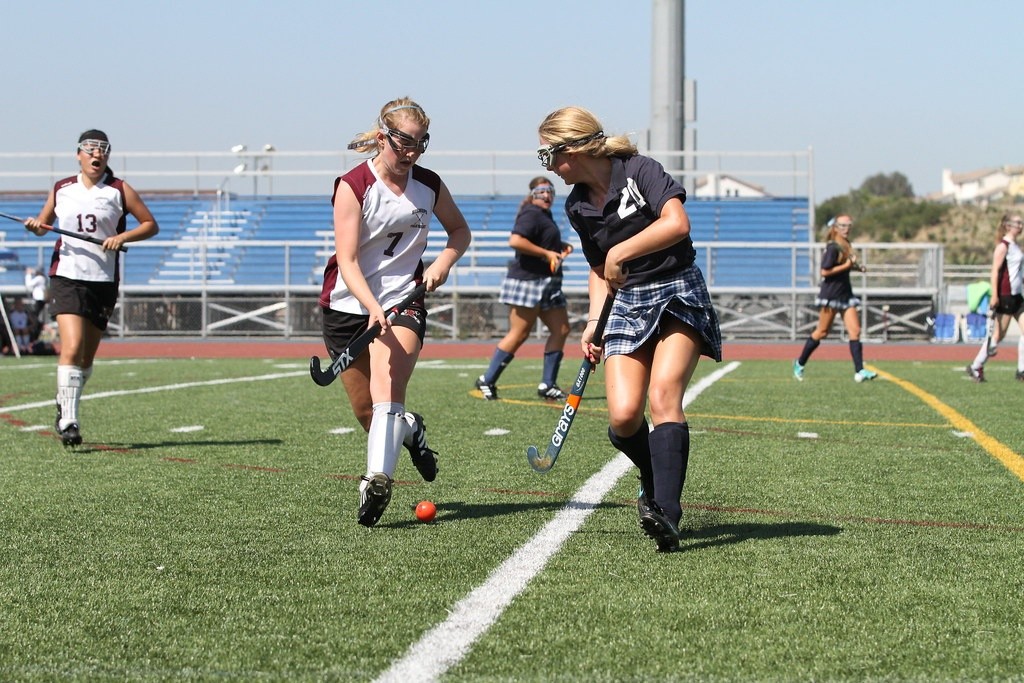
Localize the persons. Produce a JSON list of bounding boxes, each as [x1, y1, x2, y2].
[[0, 265, 49, 356], [25, 130, 160, 446], [967, 211, 1024, 383], [320, 97, 471, 527], [792, 213, 878, 383], [537, 106, 722, 549], [475, 176, 574, 401]]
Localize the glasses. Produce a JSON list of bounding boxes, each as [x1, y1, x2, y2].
[[76, 139, 114, 156], [535, 129, 607, 167], [1006, 220, 1022, 227], [386, 126, 432, 155], [531, 185, 556, 198], [838, 223, 853, 230]]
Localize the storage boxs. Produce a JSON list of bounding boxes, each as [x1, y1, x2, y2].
[[967, 294, 990, 338], [933, 313, 955, 339]]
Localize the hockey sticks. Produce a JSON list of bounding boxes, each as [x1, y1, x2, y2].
[[550, 246, 571, 274], [0, 210, 130, 255], [526, 263, 628, 472], [308, 279, 426, 389], [986, 307, 998, 357], [834, 244, 865, 273]]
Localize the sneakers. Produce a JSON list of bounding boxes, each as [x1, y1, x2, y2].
[[793, 359, 804, 382], [537, 381, 568, 400], [855, 369, 878, 383], [474, 375, 499, 401], [402, 412, 440, 482], [355, 472, 392, 528], [638, 468, 681, 548], [54, 403, 82, 445]]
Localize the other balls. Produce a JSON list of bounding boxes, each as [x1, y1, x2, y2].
[[416, 500, 437, 521]]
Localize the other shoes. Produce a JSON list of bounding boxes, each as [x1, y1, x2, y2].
[[966, 361, 986, 382], [1015, 370, 1024, 380]]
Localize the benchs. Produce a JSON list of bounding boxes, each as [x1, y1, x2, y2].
[[0, 194, 810, 287]]
